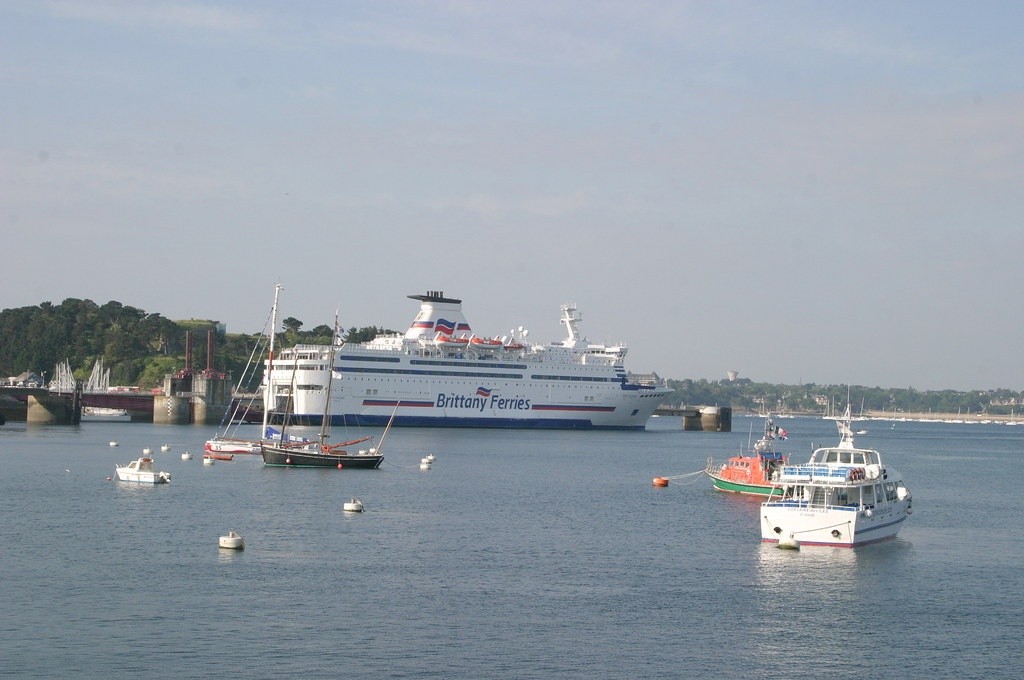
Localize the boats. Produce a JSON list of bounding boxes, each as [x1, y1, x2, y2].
[[471, 336, 502, 349], [436, 334, 470, 347], [760, 400, 913, 547], [115, 457, 171, 483], [503, 342, 525, 352]]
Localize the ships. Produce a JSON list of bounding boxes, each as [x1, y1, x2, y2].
[[258, 290, 677, 430]]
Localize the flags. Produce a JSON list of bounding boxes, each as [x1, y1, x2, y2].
[[774, 426, 787, 441]]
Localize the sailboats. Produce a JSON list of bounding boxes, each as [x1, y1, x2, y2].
[[204, 279, 348, 454], [260, 311, 401, 470], [822, 384, 869, 421], [704, 408, 805, 499]]
